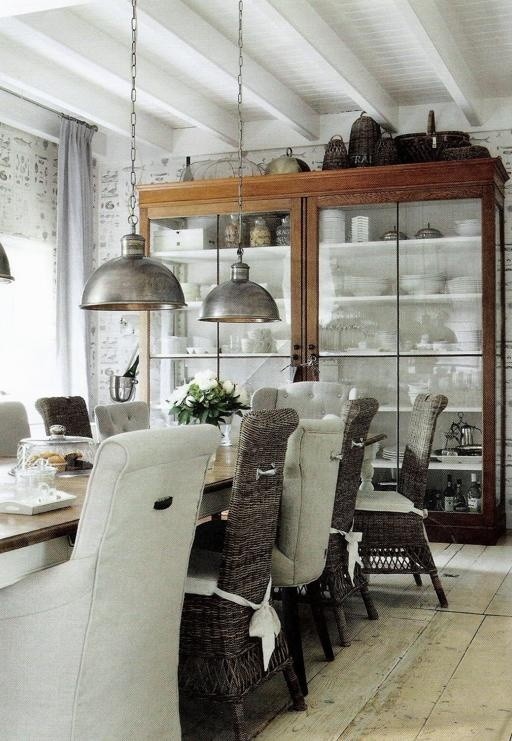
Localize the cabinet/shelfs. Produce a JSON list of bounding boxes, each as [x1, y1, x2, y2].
[[301, 157, 509, 546], [131, 176, 305, 433]]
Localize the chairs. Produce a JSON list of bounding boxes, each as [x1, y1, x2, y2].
[[182, 407, 308, 740], [0, 422, 223, 741], [353, 392, 449, 610], [0, 400, 31, 456], [253, 381, 360, 418], [33, 395, 94, 439], [323, 397, 379, 645], [94, 399, 151, 442], [270, 413, 344, 695]]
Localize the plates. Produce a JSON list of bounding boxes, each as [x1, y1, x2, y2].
[[382, 447, 405, 461], [378, 330, 482, 351], [345, 277, 388, 296], [351, 216, 368, 242], [446, 277, 482, 293], [453, 219, 482, 236], [7, 466, 92, 479], [179, 283, 218, 301], [319, 209, 345, 244]]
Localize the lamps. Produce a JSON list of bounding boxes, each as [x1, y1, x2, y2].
[[0, 241, 15, 285], [199, 0, 281, 323], [78, 0, 187, 312]]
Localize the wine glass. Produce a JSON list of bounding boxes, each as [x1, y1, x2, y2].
[[319, 311, 378, 351], [435, 370, 483, 408]]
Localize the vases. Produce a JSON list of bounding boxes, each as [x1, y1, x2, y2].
[[183, 418, 217, 471]]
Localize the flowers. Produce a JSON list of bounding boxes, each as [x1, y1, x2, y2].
[[162, 370, 253, 424]]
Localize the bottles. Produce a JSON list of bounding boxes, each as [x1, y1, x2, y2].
[[225, 214, 290, 248], [183, 156, 194, 182], [432, 472, 481, 512]]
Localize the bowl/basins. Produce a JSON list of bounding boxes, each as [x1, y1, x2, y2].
[[408, 382, 429, 406], [399, 273, 446, 295], [186, 336, 255, 354]]
